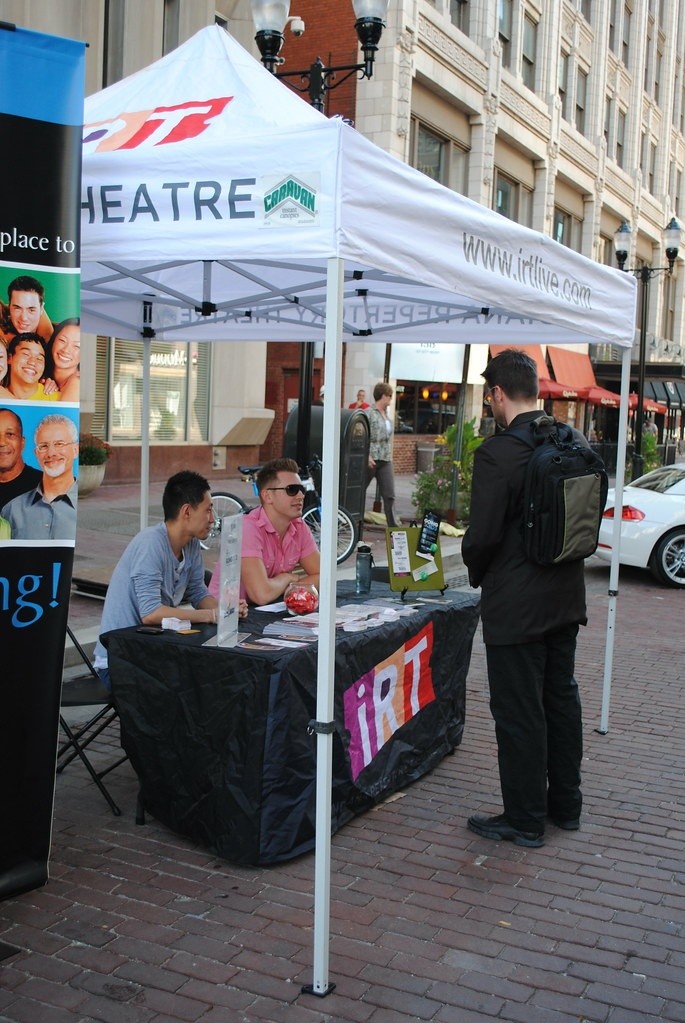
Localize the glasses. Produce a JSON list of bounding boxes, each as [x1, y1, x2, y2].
[[36, 440, 77, 453], [265, 484, 307, 496], [483, 386, 498, 405]]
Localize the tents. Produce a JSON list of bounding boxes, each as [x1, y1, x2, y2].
[[79, 23, 638, 998], [533, 377, 667, 416]]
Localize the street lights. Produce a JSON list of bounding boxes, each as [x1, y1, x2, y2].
[[248, 0, 386, 511], [613, 216, 681, 483]]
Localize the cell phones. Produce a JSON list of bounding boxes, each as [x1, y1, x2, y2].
[[136, 627, 165, 635]]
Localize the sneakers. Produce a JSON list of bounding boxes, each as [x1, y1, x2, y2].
[[549, 812, 580, 830], [467, 811, 546, 847]]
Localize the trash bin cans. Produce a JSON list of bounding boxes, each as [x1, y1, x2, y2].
[[282, 406, 371, 541]]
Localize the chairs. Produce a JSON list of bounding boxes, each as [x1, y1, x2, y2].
[[56, 626, 128, 815]]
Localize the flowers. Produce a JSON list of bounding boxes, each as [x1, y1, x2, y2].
[[79, 432, 113, 466]]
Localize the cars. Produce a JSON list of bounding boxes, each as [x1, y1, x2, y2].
[[592, 462, 685, 589]]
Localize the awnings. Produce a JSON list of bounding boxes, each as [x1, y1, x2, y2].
[[644, 381, 685, 409]]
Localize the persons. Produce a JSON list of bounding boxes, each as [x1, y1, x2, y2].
[[209, 458, 320, 618], [461, 349, 586, 847], [94, 471, 247, 695], [0, 409, 78, 541], [0, 276, 79, 403], [315, 382, 397, 533], [642, 418, 658, 453]]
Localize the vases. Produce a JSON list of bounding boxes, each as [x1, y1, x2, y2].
[[78, 464, 105, 499]]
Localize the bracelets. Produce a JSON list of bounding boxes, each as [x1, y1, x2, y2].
[[212, 609, 218, 626]]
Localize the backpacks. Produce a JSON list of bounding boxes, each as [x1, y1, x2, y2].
[[488, 415, 609, 566]]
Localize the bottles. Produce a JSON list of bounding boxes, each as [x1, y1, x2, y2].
[[355, 546, 373, 594], [283, 581, 319, 615]]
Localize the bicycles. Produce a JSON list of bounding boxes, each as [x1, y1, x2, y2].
[[193, 453, 358, 565]]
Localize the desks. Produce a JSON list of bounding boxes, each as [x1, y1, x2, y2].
[[99, 580, 482, 867]]
